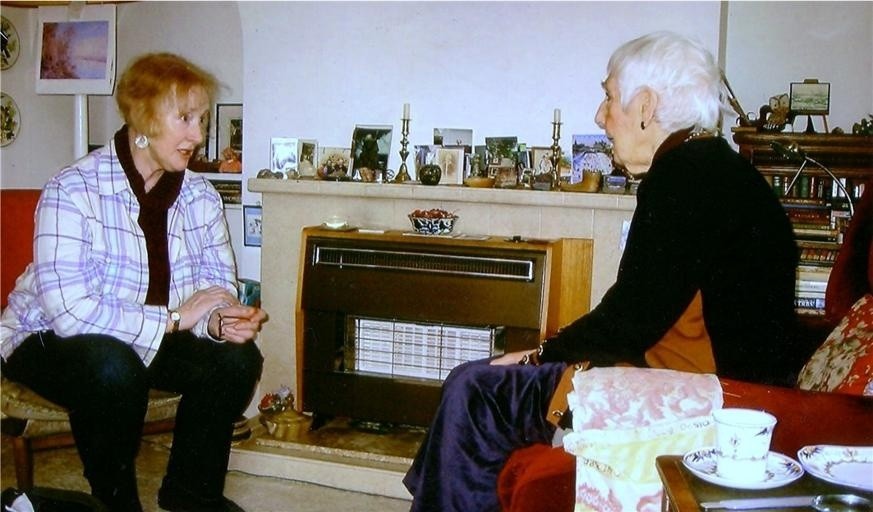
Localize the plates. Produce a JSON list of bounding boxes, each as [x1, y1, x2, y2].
[[797, 444, 873, 494], [681, 446, 805, 491]]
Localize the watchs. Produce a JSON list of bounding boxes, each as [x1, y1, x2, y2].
[[166, 307, 181, 335]]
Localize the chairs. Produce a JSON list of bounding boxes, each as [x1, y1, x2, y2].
[[0, 186, 253, 510], [495, 173, 873, 511]]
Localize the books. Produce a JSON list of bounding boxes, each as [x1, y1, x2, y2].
[[765, 172, 866, 318]]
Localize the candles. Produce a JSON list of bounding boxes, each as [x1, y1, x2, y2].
[[553, 109, 561, 124], [403, 102, 410, 121]]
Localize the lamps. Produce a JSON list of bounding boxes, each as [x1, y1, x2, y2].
[[769, 137, 855, 220]]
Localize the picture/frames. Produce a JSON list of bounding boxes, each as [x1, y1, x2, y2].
[[215, 101, 243, 163], [33, 3, 117, 97], [268, 124, 561, 190], [242, 204, 262, 248], [788, 82, 831, 116], [197, 112, 210, 160]]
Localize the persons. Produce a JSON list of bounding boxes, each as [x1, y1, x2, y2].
[[297, 141, 317, 162], [0, 54, 269, 511], [399, 30, 803, 511], [335, 157, 347, 174], [323, 159, 333, 175], [229, 119, 244, 152]]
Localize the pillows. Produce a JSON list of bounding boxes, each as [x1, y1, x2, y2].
[[795, 294, 873, 397]]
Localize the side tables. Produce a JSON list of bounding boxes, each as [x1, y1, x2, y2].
[[655, 453, 873, 510]]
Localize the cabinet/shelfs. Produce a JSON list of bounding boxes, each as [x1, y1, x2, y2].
[[730, 130, 872, 316]]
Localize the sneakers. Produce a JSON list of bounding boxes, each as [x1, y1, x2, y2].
[[158, 483, 244, 512]]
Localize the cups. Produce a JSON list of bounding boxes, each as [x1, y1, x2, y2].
[[709, 409, 777, 480]]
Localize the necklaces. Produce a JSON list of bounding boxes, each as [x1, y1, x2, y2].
[[684, 130, 706, 143]]
